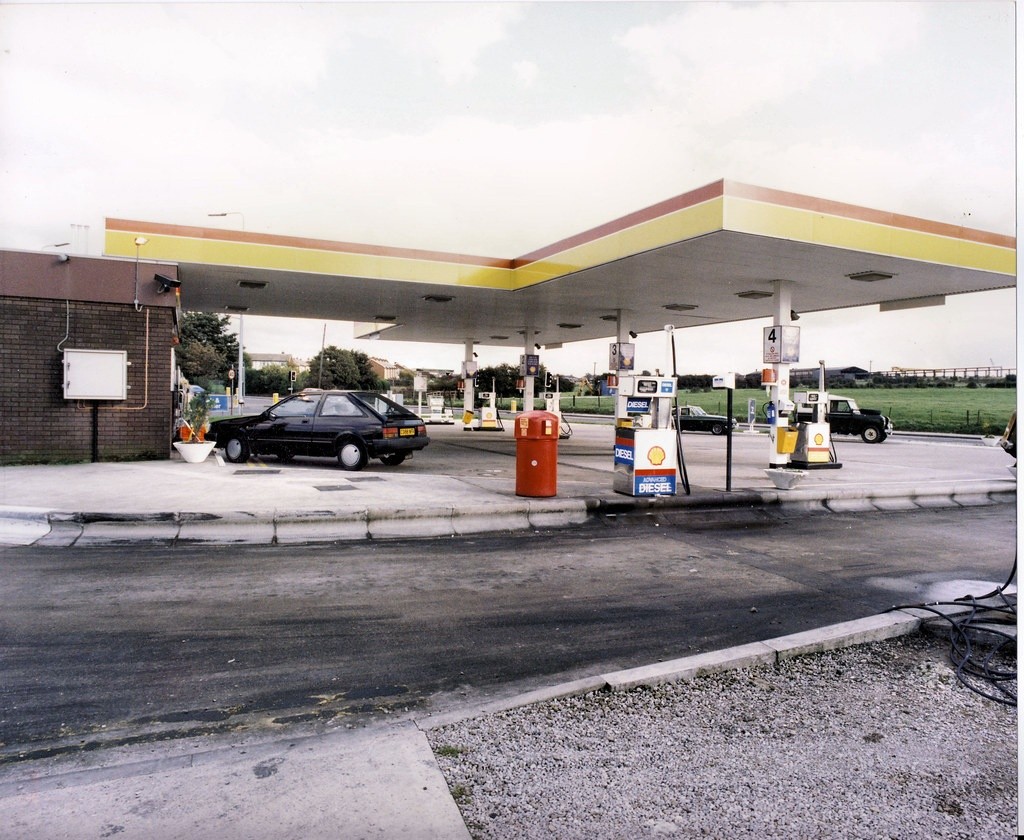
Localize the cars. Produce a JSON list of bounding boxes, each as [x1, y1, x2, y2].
[[204, 392, 431, 471], [672, 406, 739, 435]]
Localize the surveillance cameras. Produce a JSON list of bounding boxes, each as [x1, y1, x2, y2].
[[153, 274, 182, 288]]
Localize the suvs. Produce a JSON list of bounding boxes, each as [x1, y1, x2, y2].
[[797, 395, 893, 444]]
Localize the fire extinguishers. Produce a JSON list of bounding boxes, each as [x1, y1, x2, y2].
[[762, 401, 776, 424]]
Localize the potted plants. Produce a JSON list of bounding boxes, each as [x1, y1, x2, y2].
[[172, 392, 217, 463]]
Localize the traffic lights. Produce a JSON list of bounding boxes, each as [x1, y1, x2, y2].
[[291, 372, 296, 381]]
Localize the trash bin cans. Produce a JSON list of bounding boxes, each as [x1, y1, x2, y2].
[[778, 426, 799, 454], [462, 410, 474, 424], [617, 417, 633, 428], [514, 409, 559, 497]]
